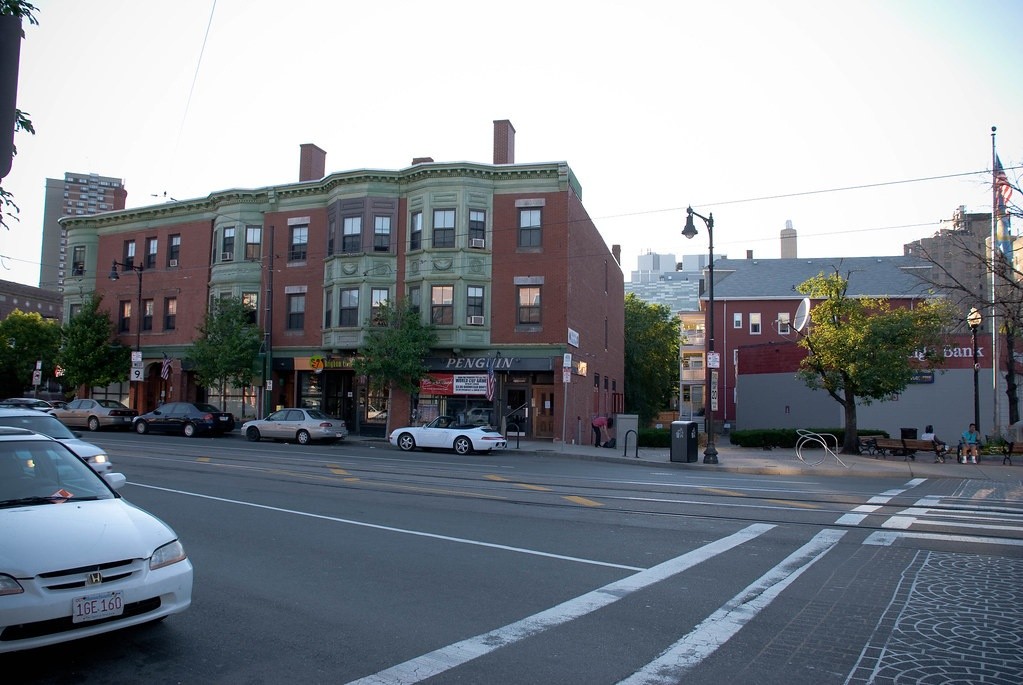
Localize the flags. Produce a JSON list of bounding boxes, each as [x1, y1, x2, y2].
[[994, 152, 1012, 227], [485, 363, 495, 402], [160, 356, 172, 380]]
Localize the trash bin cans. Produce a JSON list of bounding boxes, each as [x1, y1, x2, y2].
[[901, 428, 917, 439], [669, 421, 698, 462]]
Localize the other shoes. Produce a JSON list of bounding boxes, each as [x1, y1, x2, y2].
[[936, 459, 943, 463], [962, 459, 968, 463], [972, 459, 976, 464]]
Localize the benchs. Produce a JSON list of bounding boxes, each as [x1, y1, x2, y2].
[[858, 435, 948, 463], [1002, 442, 1023, 464]]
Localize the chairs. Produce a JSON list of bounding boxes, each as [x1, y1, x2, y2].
[[0, 459, 31, 499]]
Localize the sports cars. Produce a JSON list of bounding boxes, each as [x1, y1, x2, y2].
[[389, 416, 508, 455]]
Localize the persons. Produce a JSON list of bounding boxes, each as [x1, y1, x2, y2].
[[591, 417, 613, 448], [960, 423, 980, 464], [921, 425, 946, 463]]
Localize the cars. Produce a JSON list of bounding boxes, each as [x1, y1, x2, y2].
[[0, 425, 194, 653], [132, 401, 236, 437], [240, 408, 349, 445], [0, 405, 14, 409], [3, 398, 53, 414], [466, 408, 493, 425], [0, 408, 113, 478], [47, 400, 67, 409], [47, 398, 137, 431]]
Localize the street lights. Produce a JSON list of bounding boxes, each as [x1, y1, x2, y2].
[[682, 205, 719, 464], [967, 308, 982, 446], [108, 259, 144, 410]]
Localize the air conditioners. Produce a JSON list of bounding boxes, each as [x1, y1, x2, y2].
[[221, 251, 233, 261], [467, 316, 486, 325], [169, 260, 177, 267], [469, 238, 485, 248]]
